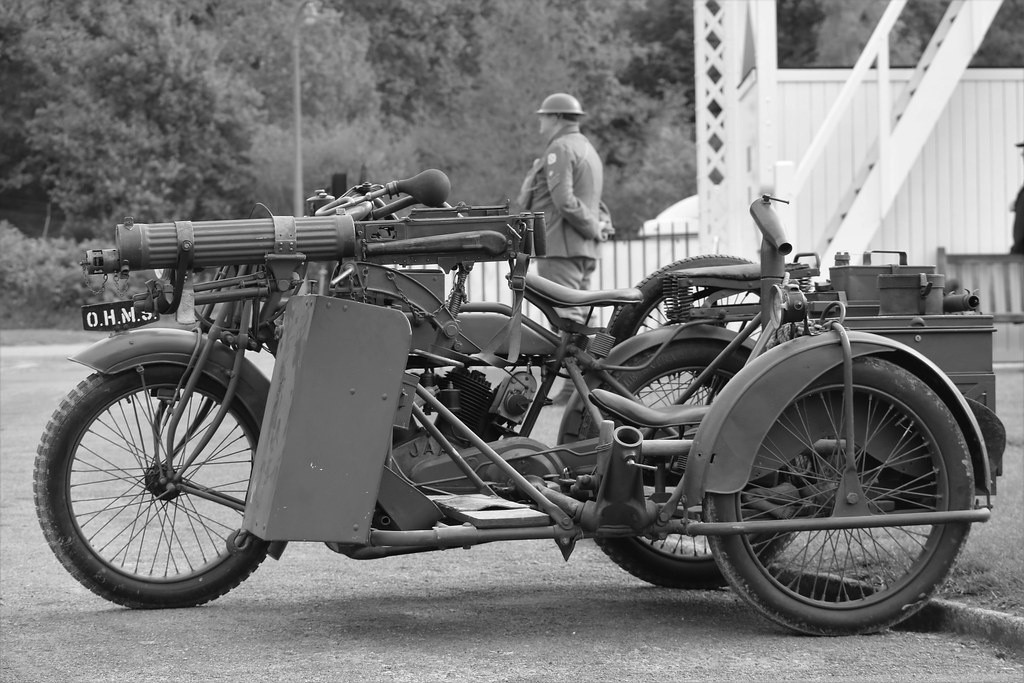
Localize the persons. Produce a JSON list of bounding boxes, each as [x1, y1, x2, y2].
[[1008, 143, 1024, 255], [518, 92, 614, 328]]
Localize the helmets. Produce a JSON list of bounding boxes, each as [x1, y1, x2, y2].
[[537, 93, 587, 114]]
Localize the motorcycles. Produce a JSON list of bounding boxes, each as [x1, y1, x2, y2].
[[34, 167, 1006, 636]]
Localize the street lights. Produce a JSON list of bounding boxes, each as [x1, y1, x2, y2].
[[290, 15, 315, 217]]
